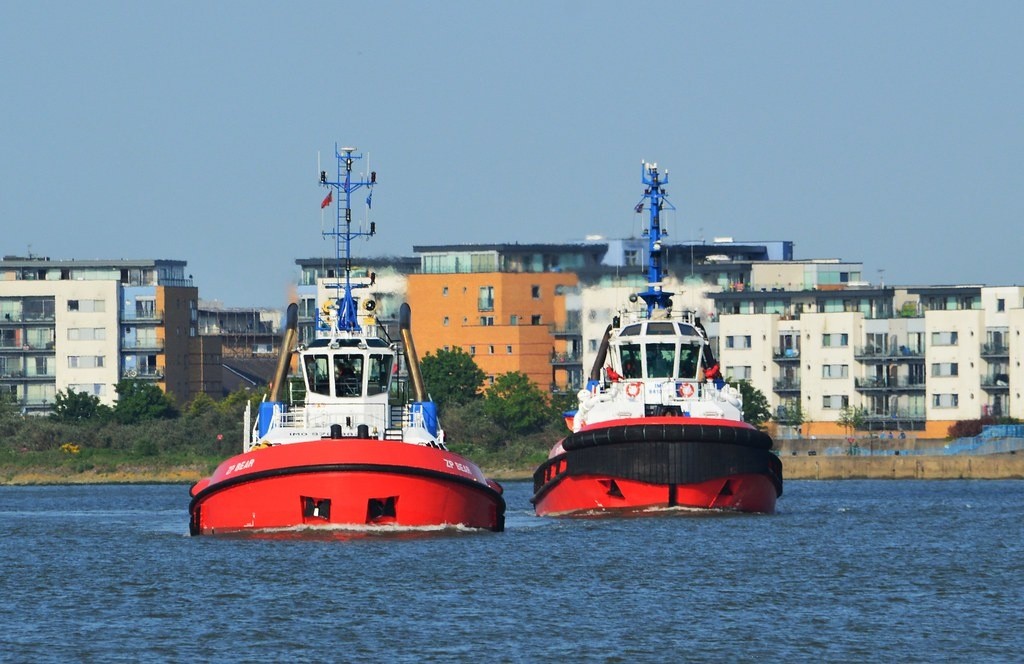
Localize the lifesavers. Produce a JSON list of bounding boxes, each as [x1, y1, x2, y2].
[[679, 383, 694, 398], [625, 382, 640, 398]]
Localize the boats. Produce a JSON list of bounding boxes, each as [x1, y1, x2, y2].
[[527, 160, 785, 513], [185, 144, 511, 535]]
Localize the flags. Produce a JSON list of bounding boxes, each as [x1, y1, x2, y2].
[[367, 188, 372, 208], [321, 192, 332, 208]]
